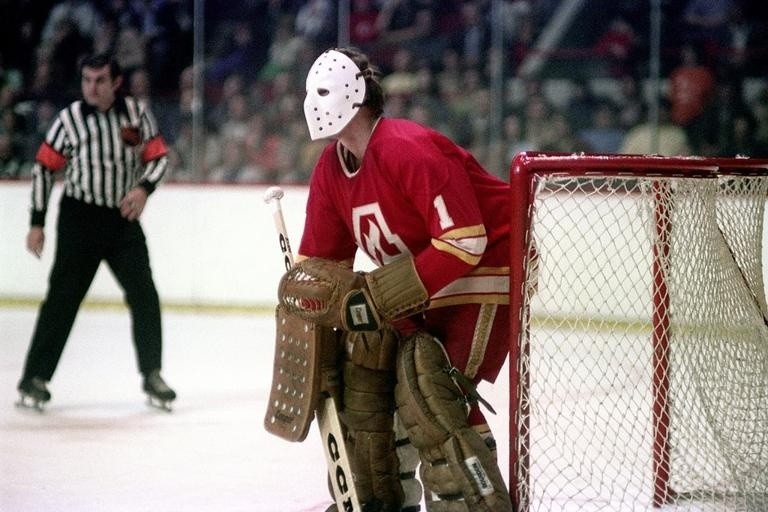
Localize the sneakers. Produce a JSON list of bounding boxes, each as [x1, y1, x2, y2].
[[143, 368, 175, 401], [17, 377, 50, 401]]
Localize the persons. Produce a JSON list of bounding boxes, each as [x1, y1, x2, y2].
[[0, 1, 768, 192], [266, 43, 517, 511], [18, 48, 178, 410]]
